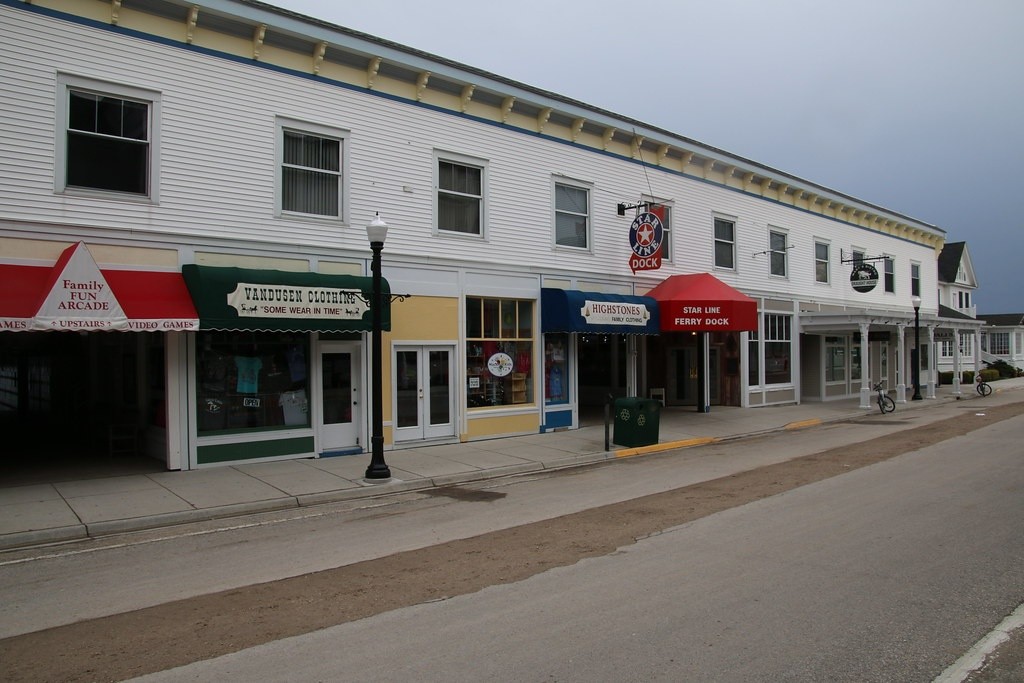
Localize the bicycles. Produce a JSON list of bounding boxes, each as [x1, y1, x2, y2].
[[873, 381, 896, 415], [976, 370, 992, 396]]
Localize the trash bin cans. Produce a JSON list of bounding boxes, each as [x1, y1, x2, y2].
[[613, 397, 660, 448]]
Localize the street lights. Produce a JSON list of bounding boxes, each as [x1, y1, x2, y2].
[[912, 295, 922, 400], [366, 210, 391, 479]]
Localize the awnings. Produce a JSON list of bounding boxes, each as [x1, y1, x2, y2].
[[0, 264, 199, 331], [182, 264, 392, 332], [541, 288, 660, 335], [645, 274, 758, 331]]
